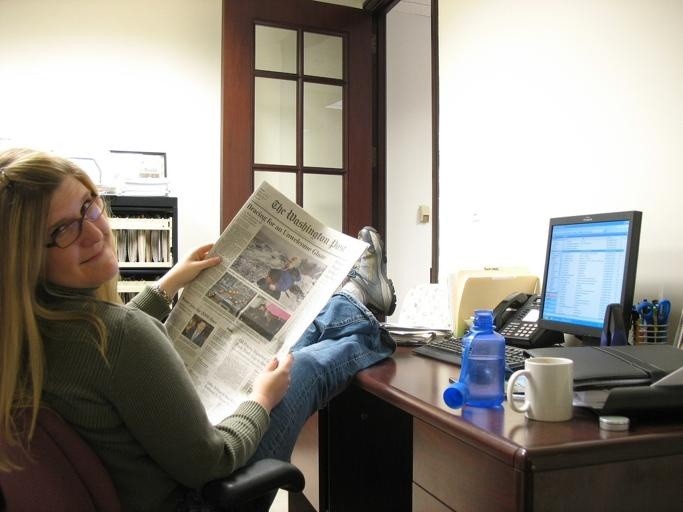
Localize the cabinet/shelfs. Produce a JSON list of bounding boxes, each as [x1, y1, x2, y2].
[[101, 194, 178, 313]]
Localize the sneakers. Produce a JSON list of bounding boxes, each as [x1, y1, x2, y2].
[[348, 225, 396, 316]]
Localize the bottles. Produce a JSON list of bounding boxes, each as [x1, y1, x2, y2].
[[442, 309, 506, 409]]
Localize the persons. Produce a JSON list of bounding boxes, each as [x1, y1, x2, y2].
[[1, 146, 398, 511]]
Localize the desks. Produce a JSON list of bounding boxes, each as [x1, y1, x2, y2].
[[289, 339, 682, 512]]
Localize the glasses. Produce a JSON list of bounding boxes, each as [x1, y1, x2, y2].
[[43, 193, 106, 249]]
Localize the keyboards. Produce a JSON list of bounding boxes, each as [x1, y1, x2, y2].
[[412, 337, 530, 380]]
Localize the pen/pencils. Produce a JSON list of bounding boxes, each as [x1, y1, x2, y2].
[[632, 300, 659, 345]]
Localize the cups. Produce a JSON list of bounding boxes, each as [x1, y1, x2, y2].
[[507, 357, 573, 422]]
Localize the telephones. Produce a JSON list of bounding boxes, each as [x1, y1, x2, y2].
[[493, 292, 553, 348]]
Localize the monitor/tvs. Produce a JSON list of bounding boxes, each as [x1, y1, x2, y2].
[[537, 211, 642, 348]]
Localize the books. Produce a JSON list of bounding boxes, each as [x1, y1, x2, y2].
[[110, 208, 172, 264], [523, 343, 682, 390]]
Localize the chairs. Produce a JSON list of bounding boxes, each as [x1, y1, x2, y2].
[[0, 390, 304, 511]]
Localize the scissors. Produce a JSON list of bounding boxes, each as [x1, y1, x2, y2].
[[638, 299, 671, 340]]
